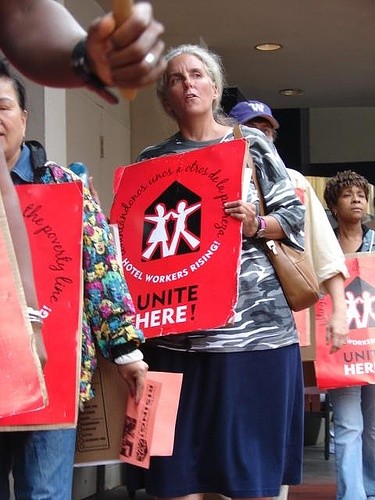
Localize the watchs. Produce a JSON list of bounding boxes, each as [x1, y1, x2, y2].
[[254, 216, 267, 240]]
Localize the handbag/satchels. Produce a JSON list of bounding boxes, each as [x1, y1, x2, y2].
[[263, 238, 319, 312]]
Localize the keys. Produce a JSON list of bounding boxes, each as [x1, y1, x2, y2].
[[266, 240, 277, 255]]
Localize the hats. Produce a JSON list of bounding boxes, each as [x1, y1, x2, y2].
[[228, 99, 281, 129]]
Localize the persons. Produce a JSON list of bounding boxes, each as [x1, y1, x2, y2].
[[0, 0, 349, 500], [323, 170, 375, 500]]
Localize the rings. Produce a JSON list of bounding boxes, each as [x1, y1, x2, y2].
[[144, 53, 158, 70]]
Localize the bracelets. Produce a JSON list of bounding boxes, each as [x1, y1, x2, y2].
[[71, 37, 119, 107]]
